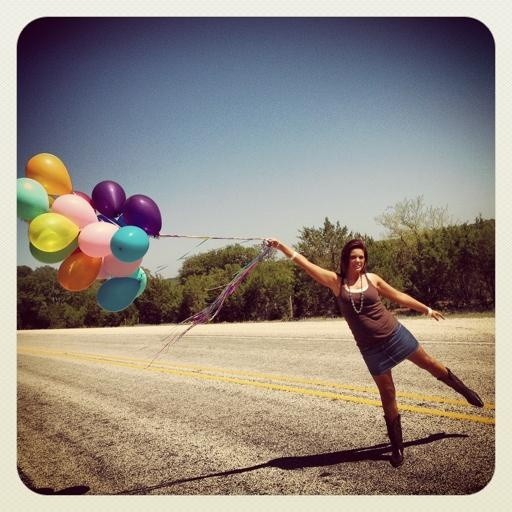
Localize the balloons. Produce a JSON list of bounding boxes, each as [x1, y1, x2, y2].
[[18, 152, 161, 313]]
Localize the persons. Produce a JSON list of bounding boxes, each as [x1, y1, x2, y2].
[[266, 237, 484, 468]]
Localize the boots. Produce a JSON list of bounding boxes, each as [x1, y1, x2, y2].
[[437, 367, 484, 407], [384, 415, 405, 466]]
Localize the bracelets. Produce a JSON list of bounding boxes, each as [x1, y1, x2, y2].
[[289, 252, 298, 261], [425, 305, 433, 318]]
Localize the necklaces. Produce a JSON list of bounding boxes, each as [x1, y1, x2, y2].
[[346, 273, 363, 313]]
